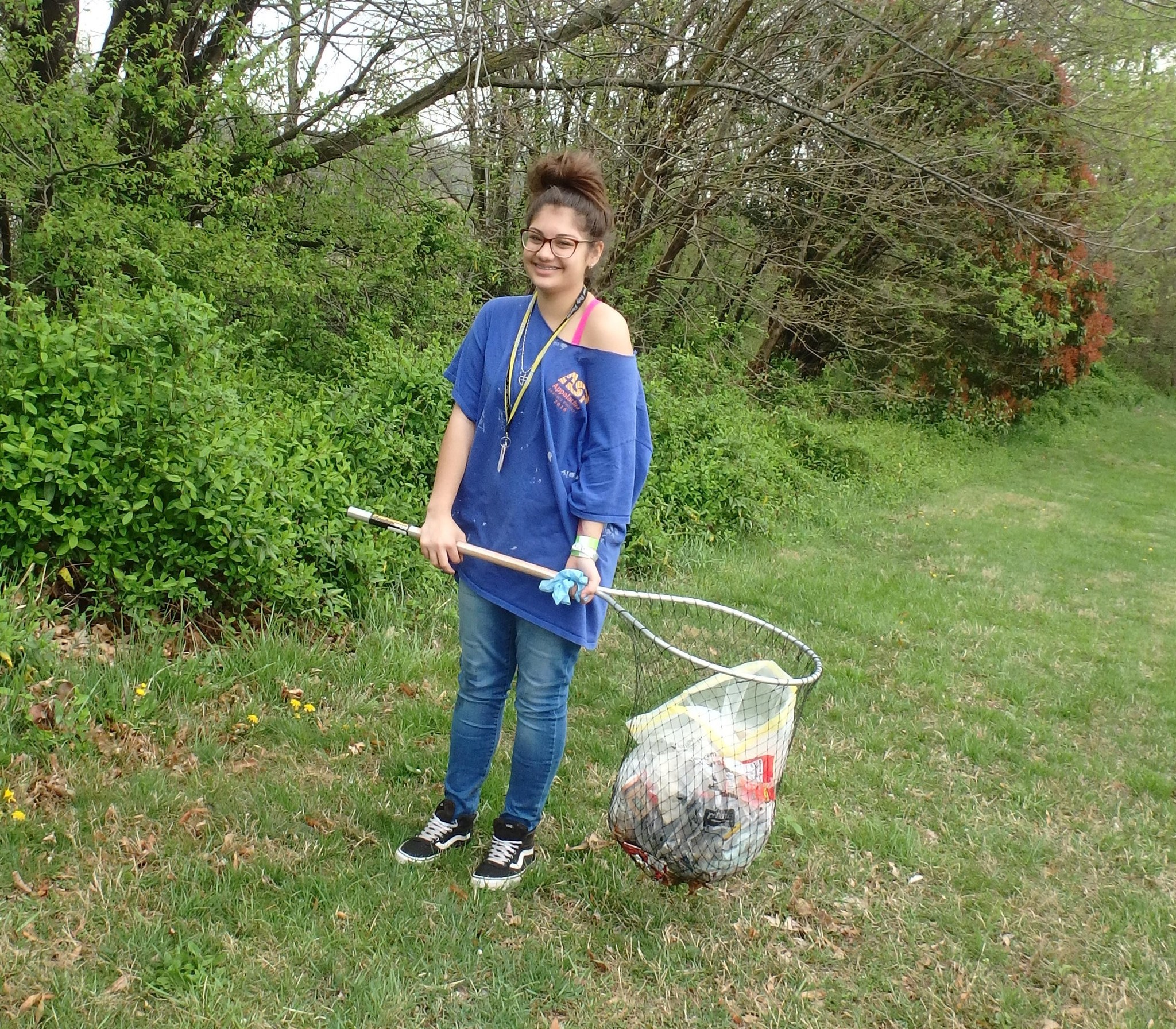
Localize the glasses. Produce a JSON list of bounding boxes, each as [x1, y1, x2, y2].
[[521, 229, 596, 259]]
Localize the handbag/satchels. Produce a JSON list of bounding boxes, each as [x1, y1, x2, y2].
[[625, 661, 798, 793]]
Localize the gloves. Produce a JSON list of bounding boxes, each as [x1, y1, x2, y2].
[[539, 569, 589, 605]]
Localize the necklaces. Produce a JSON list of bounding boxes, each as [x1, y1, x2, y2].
[[519, 312, 531, 386]]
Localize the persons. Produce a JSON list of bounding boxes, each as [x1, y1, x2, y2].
[[395, 152, 655, 890]]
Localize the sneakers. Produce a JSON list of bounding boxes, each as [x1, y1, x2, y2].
[[395, 799, 476, 864], [471, 817, 537, 890]]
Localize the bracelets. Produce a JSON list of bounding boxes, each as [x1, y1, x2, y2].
[[570, 543, 599, 563], [571, 535, 599, 558]]
